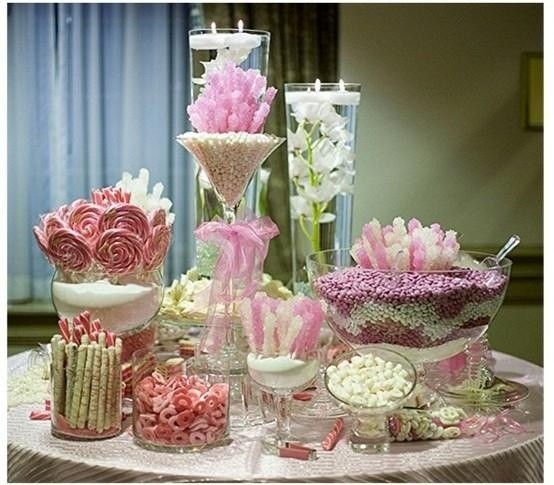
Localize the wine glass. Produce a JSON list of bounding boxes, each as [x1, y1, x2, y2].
[[244, 348, 321, 453], [435, 256, 528, 406]]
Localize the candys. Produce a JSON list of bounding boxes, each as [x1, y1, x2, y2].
[[236, 291, 328, 361], [133, 371, 229, 447], [179, 62, 278, 203], [326, 351, 412, 441], [312, 217, 506, 361], [321, 418, 343, 449], [51, 310, 123, 434], [32, 186, 170, 284]]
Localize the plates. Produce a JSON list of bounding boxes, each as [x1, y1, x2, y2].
[[156, 309, 246, 332]]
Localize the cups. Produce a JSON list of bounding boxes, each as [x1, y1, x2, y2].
[[131, 346, 232, 452], [284, 84, 363, 293], [323, 346, 419, 453], [308, 248, 514, 408], [46, 336, 124, 441], [47, 265, 166, 335], [174, 132, 286, 224]]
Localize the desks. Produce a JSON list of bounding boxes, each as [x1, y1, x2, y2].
[[8, 343, 544, 482]]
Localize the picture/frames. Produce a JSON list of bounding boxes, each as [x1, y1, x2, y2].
[[522, 51, 543, 133]]
